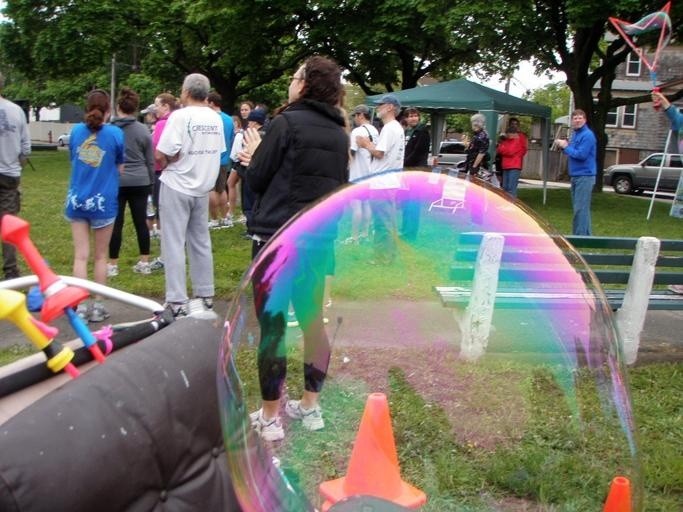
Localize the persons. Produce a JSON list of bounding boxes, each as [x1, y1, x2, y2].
[[240, 109, 268, 240], [235, 55, 351, 443], [495, 127, 522, 197], [466, 113, 493, 182], [106, 87, 151, 274], [156, 74, 227, 319], [398, 108, 430, 239], [206, 92, 232, 230], [509, 118, 528, 156], [651, 90, 683, 295], [1, 70, 31, 279], [254, 104, 270, 129], [554, 109, 597, 236], [400, 120, 408, 129], [63, 88, 127, 323], [355, 94, 405, 264], [149, 92, 180, 270], [231, 115, 241, 134], [140, 104, 161, 237], [225, 101, 255, 223], [341, 104, 379, 245]]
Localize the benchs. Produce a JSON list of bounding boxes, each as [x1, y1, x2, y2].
[[431, 230, 683, 364]]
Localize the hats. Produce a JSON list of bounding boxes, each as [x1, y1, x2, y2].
[[141, 104, 156, 114], [247, 109, 264, 123], [349, 105, 370, 116], [372, 94, 400, 108]]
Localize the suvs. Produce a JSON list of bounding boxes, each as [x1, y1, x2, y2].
[[603, 153, 683, 194], [439, 141, 469, 168]]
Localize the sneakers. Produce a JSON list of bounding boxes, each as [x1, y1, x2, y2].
[[284, 399, 324, 430], [133, 261, 151, 274], [322, 300, 332, 323], [237, 216, 247, 223], [340, 238, 358, 246], [153, 302, 188, 318], [196, 296, 214, 311], [249, 408, 285, 442], [75, 304, 90, 325], [207, 220, 220, 230], [90, 302, 110, 322], [107, 263, 117, 275], [355, 234, 370, 242], [150, 258, 164, 269], [220, 219, 229, 228], [286, 312, 299, 326]]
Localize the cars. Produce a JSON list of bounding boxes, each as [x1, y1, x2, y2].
[[57, 130, 70, 147]]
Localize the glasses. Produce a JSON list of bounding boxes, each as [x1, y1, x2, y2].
[[289, 76, 305, 81]]
